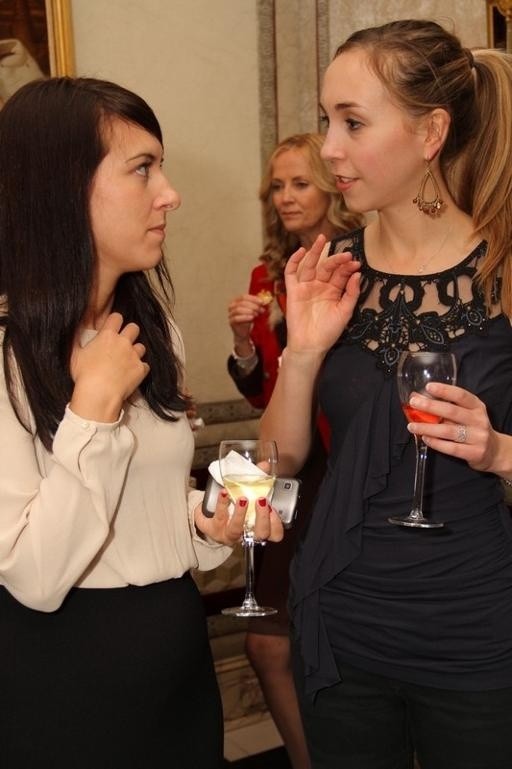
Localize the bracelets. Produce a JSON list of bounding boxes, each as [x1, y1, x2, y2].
[[232, 340, 257, 369]]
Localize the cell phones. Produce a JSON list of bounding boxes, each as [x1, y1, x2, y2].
[[201, 472, 304, 530]]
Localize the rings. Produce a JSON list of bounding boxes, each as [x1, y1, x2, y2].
[[254, 540, 267, 546], [452, 424, 466, 442]]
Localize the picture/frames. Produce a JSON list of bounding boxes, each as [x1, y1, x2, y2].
[[0, 1, 76, 104]]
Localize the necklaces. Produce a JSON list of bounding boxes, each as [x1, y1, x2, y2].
[[416, 224, 457, 275]]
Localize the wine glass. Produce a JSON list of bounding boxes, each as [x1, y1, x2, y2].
[[219, 438, 278, 616], [387, 351, 457, 527]]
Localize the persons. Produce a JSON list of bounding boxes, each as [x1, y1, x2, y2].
[[225, 132, 366, 769], [1, 73, 280, 767], [250, 16, 510, 769]]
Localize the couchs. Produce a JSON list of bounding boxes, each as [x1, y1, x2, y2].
[[188, 398, 273, 734]]
